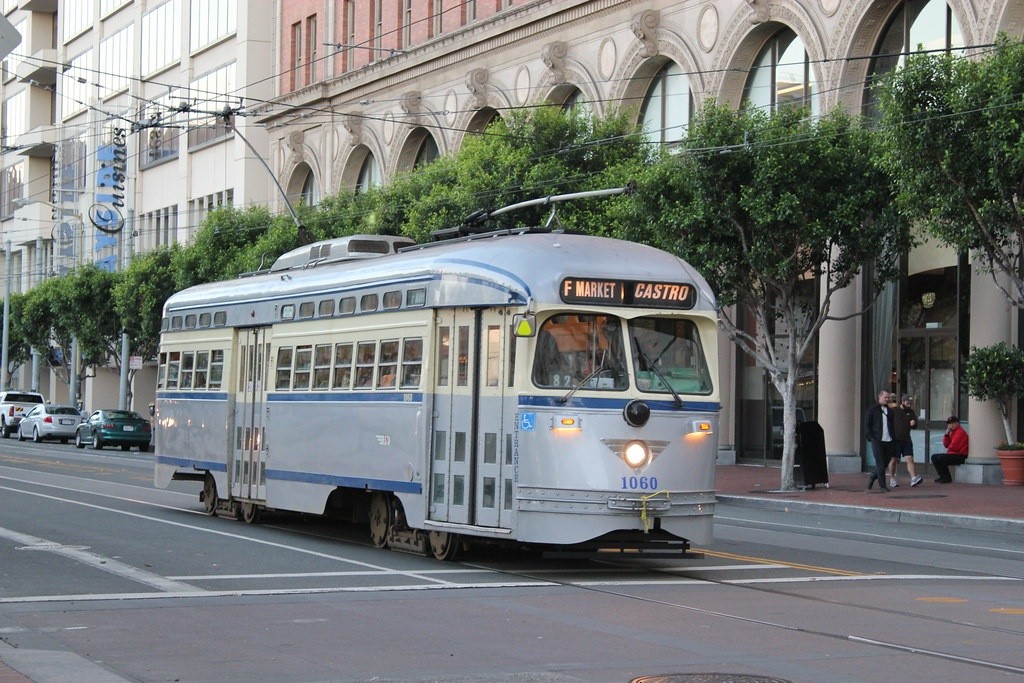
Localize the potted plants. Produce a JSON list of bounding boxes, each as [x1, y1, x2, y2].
[[959, 338, 1024, 488]]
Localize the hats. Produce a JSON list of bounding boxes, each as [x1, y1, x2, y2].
[[902, 394, 913, 403], [946, 416, 960, 423]]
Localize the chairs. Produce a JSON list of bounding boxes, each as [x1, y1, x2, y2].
[[67, 408, 75, 415], [533, 329, 568, 376]]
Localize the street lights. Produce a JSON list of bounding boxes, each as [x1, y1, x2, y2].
[[14, 197, 83, 270]]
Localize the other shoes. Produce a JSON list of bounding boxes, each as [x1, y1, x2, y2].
[[935, 478, 952, 483], [880, 488, 889, 491], [869, 475, 875, 490]]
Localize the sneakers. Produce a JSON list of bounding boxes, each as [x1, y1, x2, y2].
[[890, 482, 898, 487], [911, 477, 923, 487]]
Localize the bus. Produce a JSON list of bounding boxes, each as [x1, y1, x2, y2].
[[153, 225, 722, 565]]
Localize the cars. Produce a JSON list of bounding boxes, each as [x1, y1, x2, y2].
[[17, 403, 86, 443], [75, 409, 151, 452]]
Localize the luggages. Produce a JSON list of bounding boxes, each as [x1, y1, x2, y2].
[[795, 421, 829, 491]]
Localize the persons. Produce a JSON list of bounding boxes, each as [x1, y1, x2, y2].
[[888, 394, 923, 487], [533, 327, 565, 387], [931, 416, 969, 484], [863, 389, 890, 493], [633, 332, 672, 370]]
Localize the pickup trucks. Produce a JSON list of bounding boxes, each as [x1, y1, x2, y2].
[[0, 391, 46, 438]]
